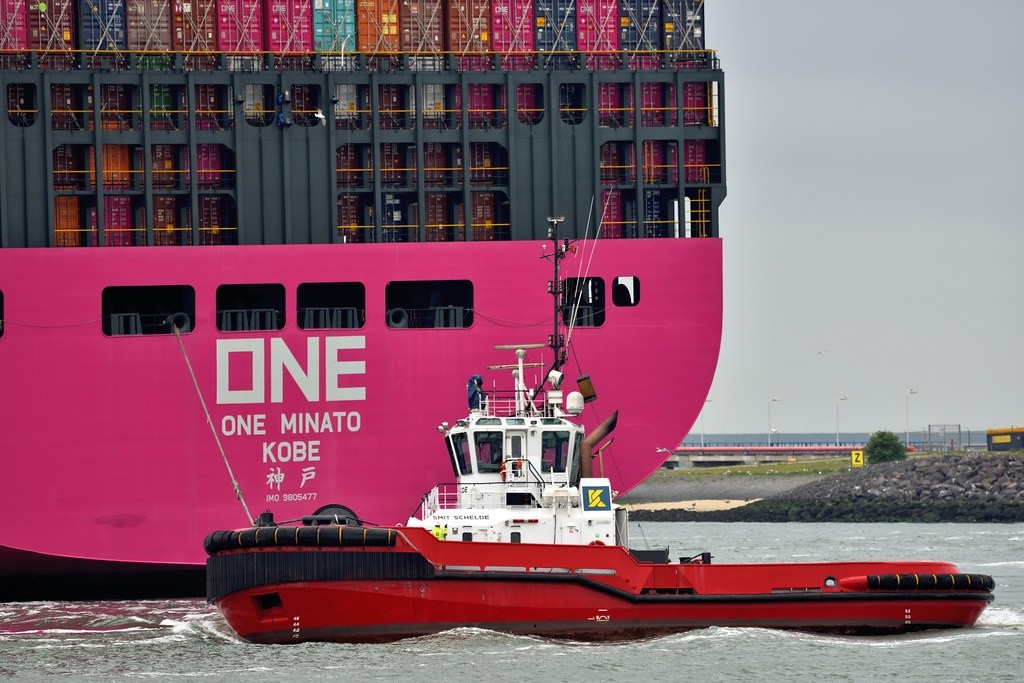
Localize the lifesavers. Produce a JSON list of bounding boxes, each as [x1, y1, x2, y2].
[[500, 462, 506, 481], [588, 540, 605, 546]]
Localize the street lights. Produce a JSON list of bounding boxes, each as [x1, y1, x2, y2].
[[906, 388, 918, 446], [767, 399, 780, 447], [836, 397, 848, 447], [701, 400, 714, 448]]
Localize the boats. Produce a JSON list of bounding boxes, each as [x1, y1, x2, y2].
[[170, 186, 996, 639]]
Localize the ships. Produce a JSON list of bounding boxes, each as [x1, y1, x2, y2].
[[0, 0, 730, 575]]
